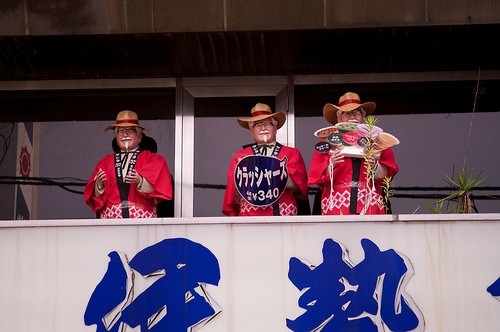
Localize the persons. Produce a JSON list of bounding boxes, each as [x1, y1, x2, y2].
[[83, 110, 172, 218], [307, 91, 400, 215], [222, 103, 309, 216]]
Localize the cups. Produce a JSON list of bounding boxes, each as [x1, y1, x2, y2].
[[314, 122, 400, 153]]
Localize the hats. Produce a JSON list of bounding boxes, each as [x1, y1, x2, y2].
[[237, 103, 286, 130], [104, 110, 146, 134], [323, 91, 376, 125]]
